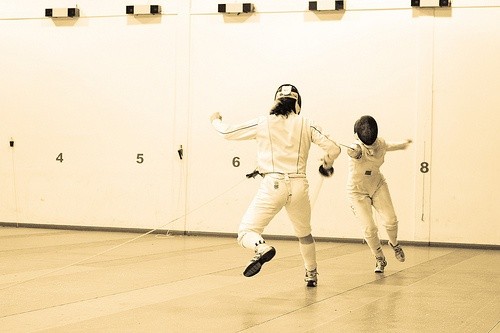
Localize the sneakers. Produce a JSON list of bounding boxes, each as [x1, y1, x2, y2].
[[305, 271, 317, 287], [374, 257, 387, 273], [242, 243, 276, 277], [388, 241, 405, 262]]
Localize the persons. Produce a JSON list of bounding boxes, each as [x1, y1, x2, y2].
[[210, 83, 341, 286], [347, 114, 413, 272]]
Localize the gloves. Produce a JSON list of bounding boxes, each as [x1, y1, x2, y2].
[[321, 154, 333, 170], [347, 144, 359, 157]]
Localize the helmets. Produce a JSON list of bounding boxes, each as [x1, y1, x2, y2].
[[274, 84, 301, 115], [353, 115, 378, 149]]
